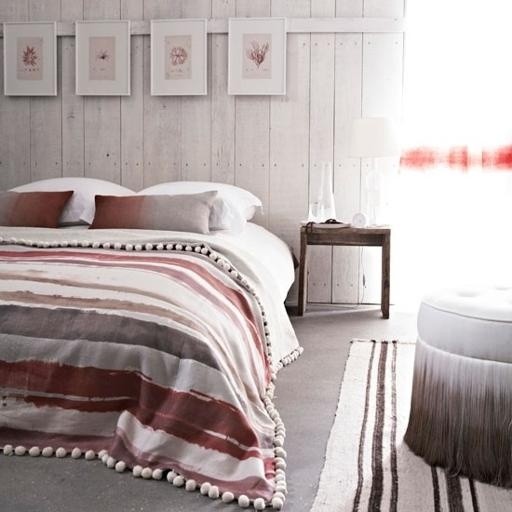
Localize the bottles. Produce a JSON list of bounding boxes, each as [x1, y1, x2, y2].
[[318, 161, 336, 221]]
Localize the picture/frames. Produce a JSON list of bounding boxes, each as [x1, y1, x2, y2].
[[3, 17, 288, 97]]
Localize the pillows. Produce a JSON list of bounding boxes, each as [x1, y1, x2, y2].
[[0, 177, 265, 236]]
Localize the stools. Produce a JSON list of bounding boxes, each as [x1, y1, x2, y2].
[[403, 284, 512, 488]]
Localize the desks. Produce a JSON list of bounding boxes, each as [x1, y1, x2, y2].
[[296, 226, 391, 319]]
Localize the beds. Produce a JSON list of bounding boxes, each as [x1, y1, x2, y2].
[[1, 220, 301, 461]]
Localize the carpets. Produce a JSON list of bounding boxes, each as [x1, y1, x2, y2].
[[309, 336, 511, 511]]
